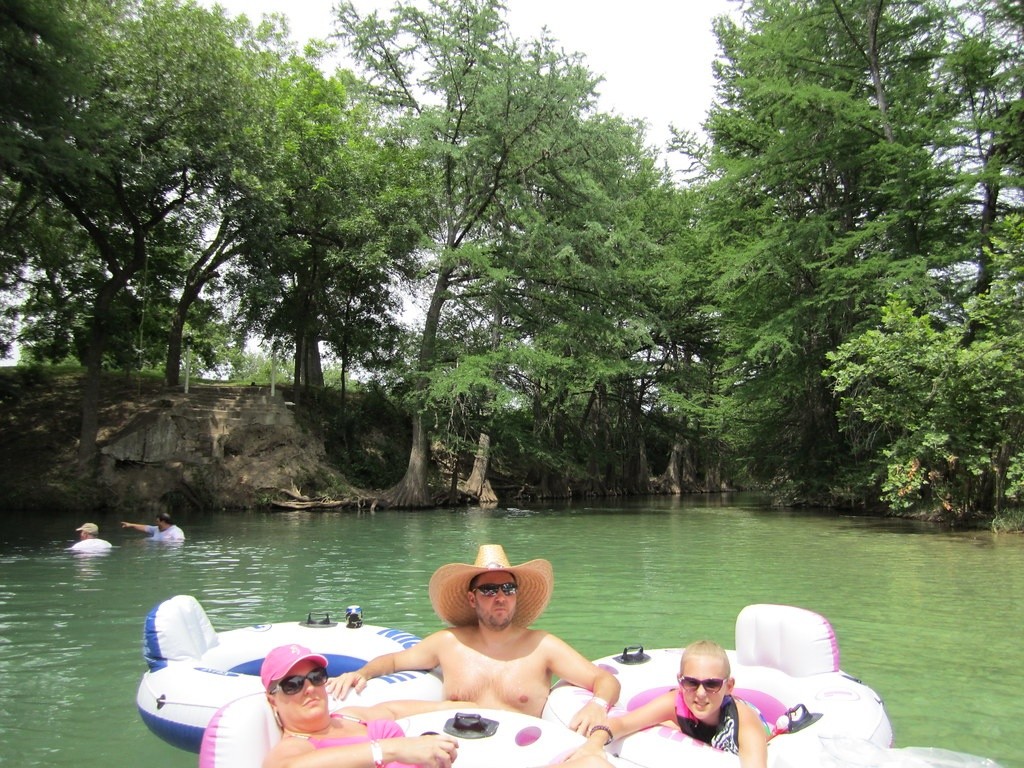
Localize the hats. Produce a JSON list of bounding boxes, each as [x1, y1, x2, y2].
[[75, 522, 99, 535], [261, 644, 328, 691], [429, 544, 553, 628]]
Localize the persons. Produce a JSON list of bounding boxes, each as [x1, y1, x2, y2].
[[261, 644, 459, 768], [64, 523, 112, 557], [120, 512, 185, 547], [562, 637, 771, 768], [323, 544, 621, 736]]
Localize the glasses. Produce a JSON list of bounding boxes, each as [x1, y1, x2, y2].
[[471, 582, 518, 597], [269, 666, 327, 696], [155, 520, 162, 524], [677, 673, 729, 693]]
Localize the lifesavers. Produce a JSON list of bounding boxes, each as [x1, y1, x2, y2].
[[135, 595, 894, 768]]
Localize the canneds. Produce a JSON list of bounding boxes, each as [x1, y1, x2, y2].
[[345, 605, 363, 628]]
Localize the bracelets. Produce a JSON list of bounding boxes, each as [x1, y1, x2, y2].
[[370, 739, 385, 768], [589, 725, 613, 746], [593, 697, 611, 710]]
